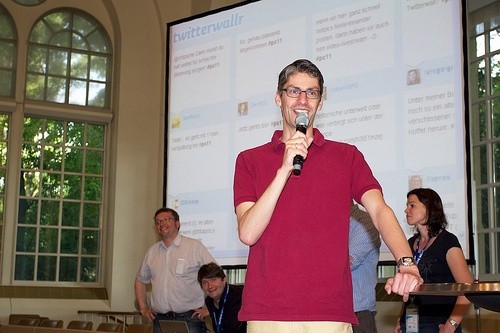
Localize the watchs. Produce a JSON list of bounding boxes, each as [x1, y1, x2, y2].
[[397, 257, 418, 273], [447, 317, 459, 328]]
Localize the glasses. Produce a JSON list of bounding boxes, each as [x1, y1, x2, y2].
[[279, 87, 323, 99], [155, 217, 177, 224]]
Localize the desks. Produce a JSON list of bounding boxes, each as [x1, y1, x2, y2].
[[408, 282, 500, 333]]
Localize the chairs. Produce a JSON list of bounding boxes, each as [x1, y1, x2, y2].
[[0, 314, 190, 333]]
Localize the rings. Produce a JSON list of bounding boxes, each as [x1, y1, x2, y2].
[[199, 314, 201, 317], [295, 143, 298, 150]]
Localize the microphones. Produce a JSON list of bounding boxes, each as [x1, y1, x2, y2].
[[293, 112, 310, 176]]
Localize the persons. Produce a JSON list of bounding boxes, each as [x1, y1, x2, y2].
[[197, 263, 247, 333], [393, 188, 475, 333], [348, 198, 382, 333], [233, 59, 423, 333], [135, 208, 222, 333]]
